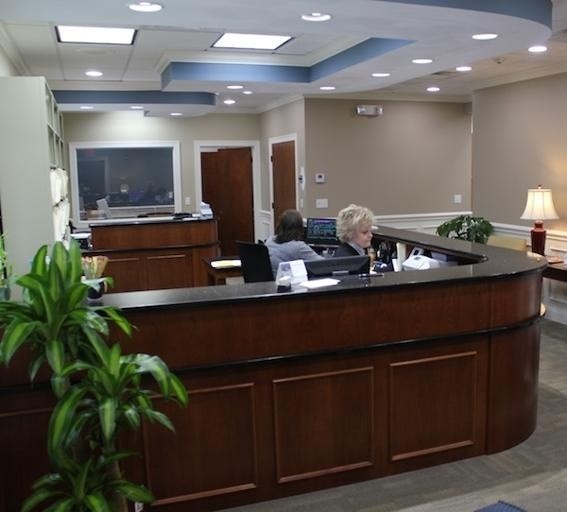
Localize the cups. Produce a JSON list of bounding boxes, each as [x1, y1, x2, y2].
[[392, 259, 402, 271]]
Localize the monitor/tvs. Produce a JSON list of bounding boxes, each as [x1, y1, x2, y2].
[[306, 218, 340, 247], [304, 255, 370, 280]]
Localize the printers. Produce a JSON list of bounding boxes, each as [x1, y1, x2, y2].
[[402, 255, 458, 270]]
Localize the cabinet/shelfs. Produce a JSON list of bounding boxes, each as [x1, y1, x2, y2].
[[2, 76, 70, 298]]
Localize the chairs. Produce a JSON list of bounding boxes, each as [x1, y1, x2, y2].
[[235, 240, 274, 283]]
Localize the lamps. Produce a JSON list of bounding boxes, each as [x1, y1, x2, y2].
[[521, 188, 558, 255]]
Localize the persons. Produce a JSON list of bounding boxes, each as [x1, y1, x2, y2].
[[112, 184, 130, 202], [265, 204, 374, 279]]
